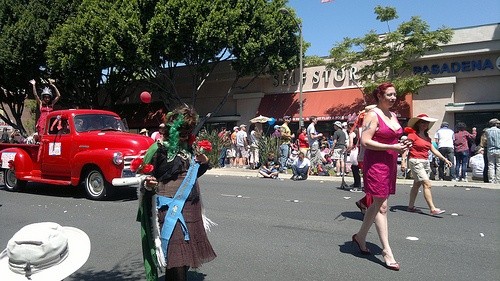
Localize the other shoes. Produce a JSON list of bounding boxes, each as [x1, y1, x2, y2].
[[350, 187, 363, 192], [336, 173, 346, 176], [431, 208, 445, 215], [440, 178, 443, 180], [349, 184, 354, 187], [456, 178, 459, 181], [451, 178, 454, 181], [272, 175, 277, 179], [407, 205, 422, 213], [461, 178, 466, 182]]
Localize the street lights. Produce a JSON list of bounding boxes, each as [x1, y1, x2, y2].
[[279, 8, 303, 130]]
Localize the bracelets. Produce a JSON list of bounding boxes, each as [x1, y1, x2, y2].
[[402, 158, 405, 161], [442, 157, 445, 161], [144, 181, 152, 191]]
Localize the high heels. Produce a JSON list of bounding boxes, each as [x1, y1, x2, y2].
[[381, 251, 400, 271], [352, 233, 370, 254]]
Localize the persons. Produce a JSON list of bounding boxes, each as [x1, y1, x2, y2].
[[469, 146, 486, 181], [11, 130, 26, 144], [258, 151, 281, 178], [352, 82, 413, 270], [30, 77, 63, 143], [435, 122, 456, 181], [139, 115, 437, 193], [486, 119, 500, 184], [291, 152, 311, 180], [1, 128, 10, 143], [135, 107, 217, 280], [481, 128, 489, 183], [400, 114, 452, 216], [0, 221, 91, 281], [453, 123, 477, 181]]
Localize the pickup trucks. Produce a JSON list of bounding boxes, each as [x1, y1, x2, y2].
[[0, 108, 157, 201]]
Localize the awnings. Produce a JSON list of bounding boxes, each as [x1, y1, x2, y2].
[[255, 88, 413, 122]]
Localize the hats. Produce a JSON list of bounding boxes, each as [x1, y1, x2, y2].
[[233, 126, 239, 130], [240, 124, 247, 128], [408, 114, 437, 130], [159, 123, 165, 128], [489, 119, 500, 126], [283, 116, 291, 119], [139, 129, 148, 134], [476, 147, 485, 153], [333, 121, 342, 129]]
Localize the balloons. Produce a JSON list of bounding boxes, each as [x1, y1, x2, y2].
[[268, 118, 275, 126], [140, 91, 151, 103]]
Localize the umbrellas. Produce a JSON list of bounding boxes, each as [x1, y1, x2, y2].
[[249, 115, 269, 129]]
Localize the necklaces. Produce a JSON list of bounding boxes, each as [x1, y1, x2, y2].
[[177, 151, 190, 159]]
